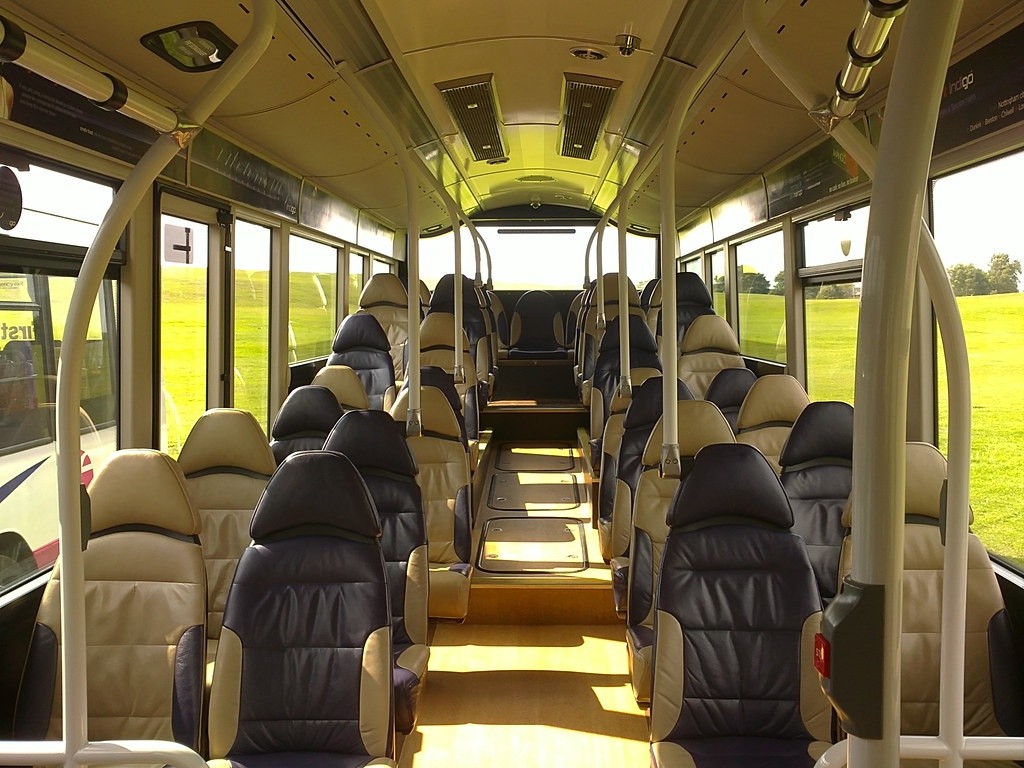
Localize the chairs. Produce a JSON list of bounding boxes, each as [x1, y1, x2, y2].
[[0, 274, 1024, 768]]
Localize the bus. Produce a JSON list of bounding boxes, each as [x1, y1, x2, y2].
[[0, 271, 115, 588]]
